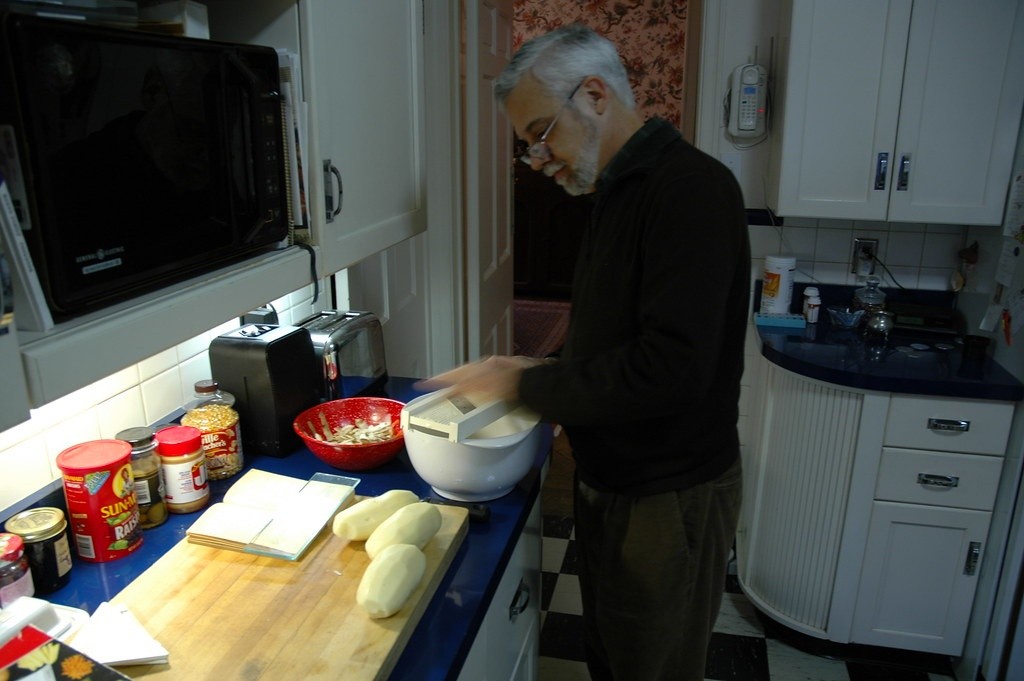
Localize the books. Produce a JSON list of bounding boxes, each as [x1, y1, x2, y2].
[[0, 600, 170, 681], [186, 467, 357, 562]]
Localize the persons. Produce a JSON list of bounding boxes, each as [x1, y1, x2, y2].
[[412, 24, 752, 681]]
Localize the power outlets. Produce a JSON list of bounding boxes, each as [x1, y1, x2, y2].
[[851, 237, 879, 275], [721, 152, 742, 182]]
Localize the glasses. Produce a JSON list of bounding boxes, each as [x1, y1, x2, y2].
[[515, 76, 588, 167]]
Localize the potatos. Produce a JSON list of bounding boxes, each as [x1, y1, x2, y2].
[[332, 490, 443, 618]]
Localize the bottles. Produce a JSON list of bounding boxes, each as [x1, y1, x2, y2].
[[0, 379, 245, 608], [802, 286, 822, 324], [759, 254, 797, 314], [852, 277, 894, 365]]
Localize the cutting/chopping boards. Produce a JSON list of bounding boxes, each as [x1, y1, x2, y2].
[[67, 492, 469, 681]]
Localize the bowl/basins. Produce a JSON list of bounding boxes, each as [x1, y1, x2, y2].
[[293, 397, 406, 471], [827, 305, 866, 329], [400, 387, 545, 503]]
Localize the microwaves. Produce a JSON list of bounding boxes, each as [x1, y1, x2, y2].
[[0, 12, 290, 324]]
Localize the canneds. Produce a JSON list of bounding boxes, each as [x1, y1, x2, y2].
[[0, 425, 210, 610]]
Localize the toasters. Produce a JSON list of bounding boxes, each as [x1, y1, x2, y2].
[[210, 309, 389, 457]]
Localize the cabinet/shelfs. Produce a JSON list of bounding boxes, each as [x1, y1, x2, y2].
[[728, 354, 1016, 676], [766, 0, 1024, 227], [210, 0, 428, 278], [484, 495, 543, 681]]
